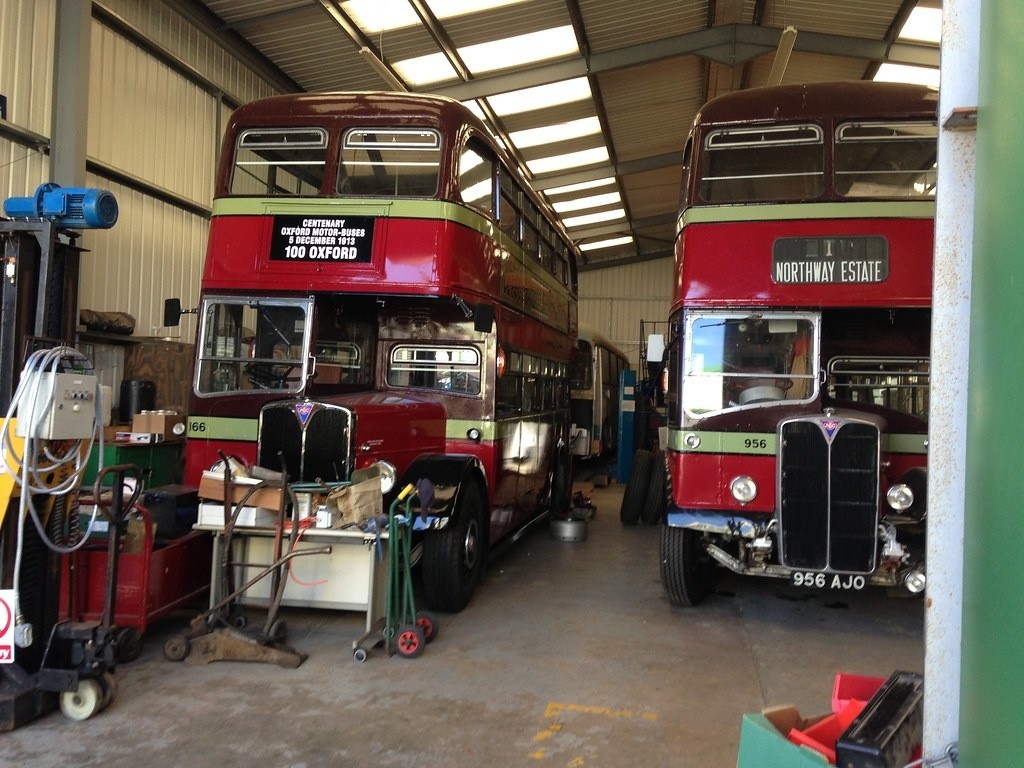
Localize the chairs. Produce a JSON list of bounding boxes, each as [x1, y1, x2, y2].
[[730, 365, 776, 397], [286, 362, 342, 393]]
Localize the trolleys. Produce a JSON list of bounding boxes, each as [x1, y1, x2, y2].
[[28, 462, 146, 722], [162, 544, 332, 664], [383, 479, 439, 659], [210, 447, 289, 652]]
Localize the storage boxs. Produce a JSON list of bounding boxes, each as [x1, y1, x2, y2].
[[198, 473, 292, 511], [198, 501, 288, 528], [132, 413, 185, 442]]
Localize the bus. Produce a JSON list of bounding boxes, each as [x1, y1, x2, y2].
[[644, 80, 936, 610], [568, 319, 631, 469], [161, 88, 588, 613]]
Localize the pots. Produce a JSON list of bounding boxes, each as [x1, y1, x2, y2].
[[117, 378, 157, 424]]
